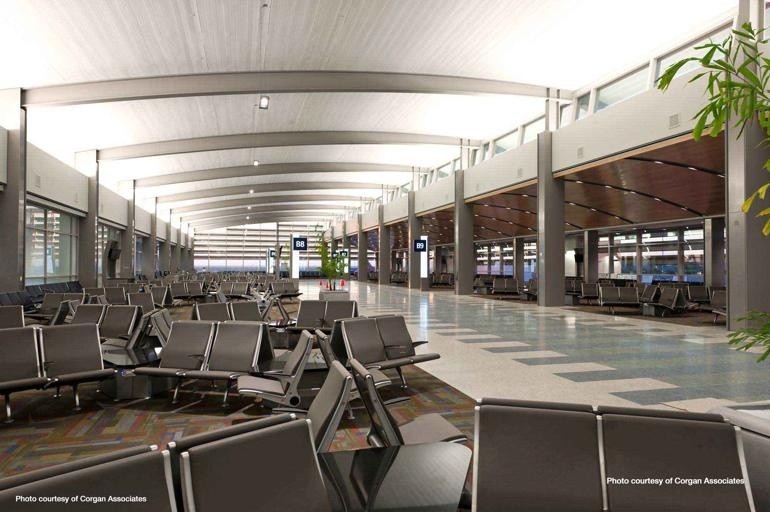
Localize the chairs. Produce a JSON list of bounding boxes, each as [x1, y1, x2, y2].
[[472, 396, 770, 512], [473, 271, 730, 327]]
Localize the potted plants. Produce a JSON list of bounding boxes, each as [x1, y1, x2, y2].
[[315, 236, 350, 301]]
